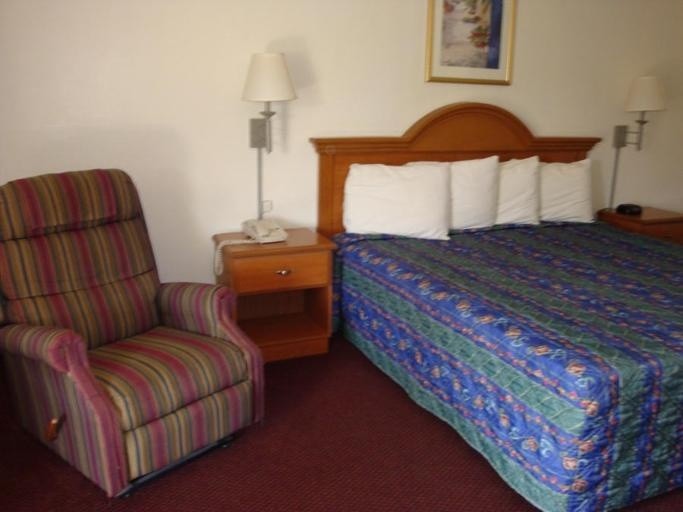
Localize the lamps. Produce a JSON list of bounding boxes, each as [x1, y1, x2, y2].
[[239, 50, 298, 153], [613, 76, 665, 148]]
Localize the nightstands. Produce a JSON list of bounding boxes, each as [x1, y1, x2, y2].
[[212, 230, 335, 360], [600, 205, 681, 238]]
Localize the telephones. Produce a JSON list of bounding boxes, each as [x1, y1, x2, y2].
[[240, 218, 287, 244]]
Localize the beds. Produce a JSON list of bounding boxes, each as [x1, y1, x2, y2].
[[308, 101, 683, 460]]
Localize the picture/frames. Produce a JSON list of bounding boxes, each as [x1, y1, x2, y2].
[[426, 1, 516, 85]]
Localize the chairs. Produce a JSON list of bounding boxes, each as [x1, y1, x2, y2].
[[1, 169, 267, 496]]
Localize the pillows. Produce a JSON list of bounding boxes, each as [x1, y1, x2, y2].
[[497, 155, 540, 229], [341, 161, 451, 241], [540, 158, 597, 225], [447, 154, 499, 232]]
